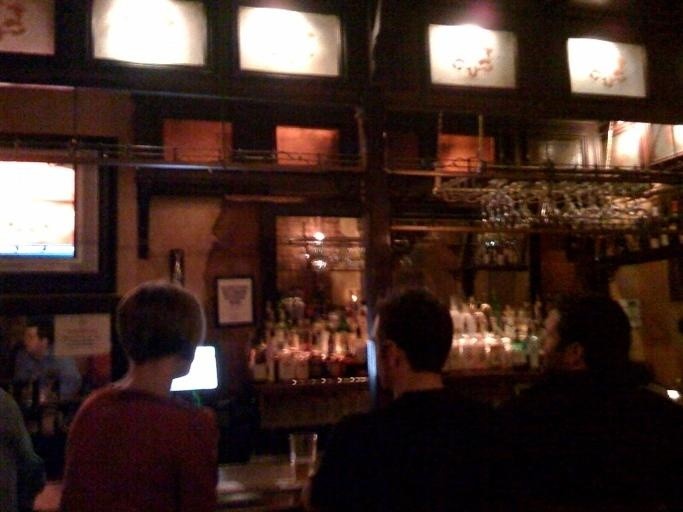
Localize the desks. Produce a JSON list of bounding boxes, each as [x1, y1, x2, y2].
[[215, 463, 321, 512]]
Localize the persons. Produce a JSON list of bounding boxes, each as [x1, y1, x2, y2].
[[296, 283, 522, 511], [11, 323, 82, 512], [488, 284, 682, 510], [0, 387, 47, 512], [54, 278, 223, 510]]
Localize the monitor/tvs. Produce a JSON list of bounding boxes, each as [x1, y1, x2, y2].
[[171, 345, 220, 392], [0, 144, 101, 275]]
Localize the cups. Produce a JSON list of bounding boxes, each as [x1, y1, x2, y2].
[[290, 431, 317, 483]]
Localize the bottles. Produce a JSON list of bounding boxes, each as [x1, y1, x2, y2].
[[250, 290, 547, 382]]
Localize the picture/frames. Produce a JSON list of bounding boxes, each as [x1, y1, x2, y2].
[[211, 272, 257, 329]]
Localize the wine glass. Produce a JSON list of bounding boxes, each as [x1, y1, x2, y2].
[[477, 178, 679, 266]]
